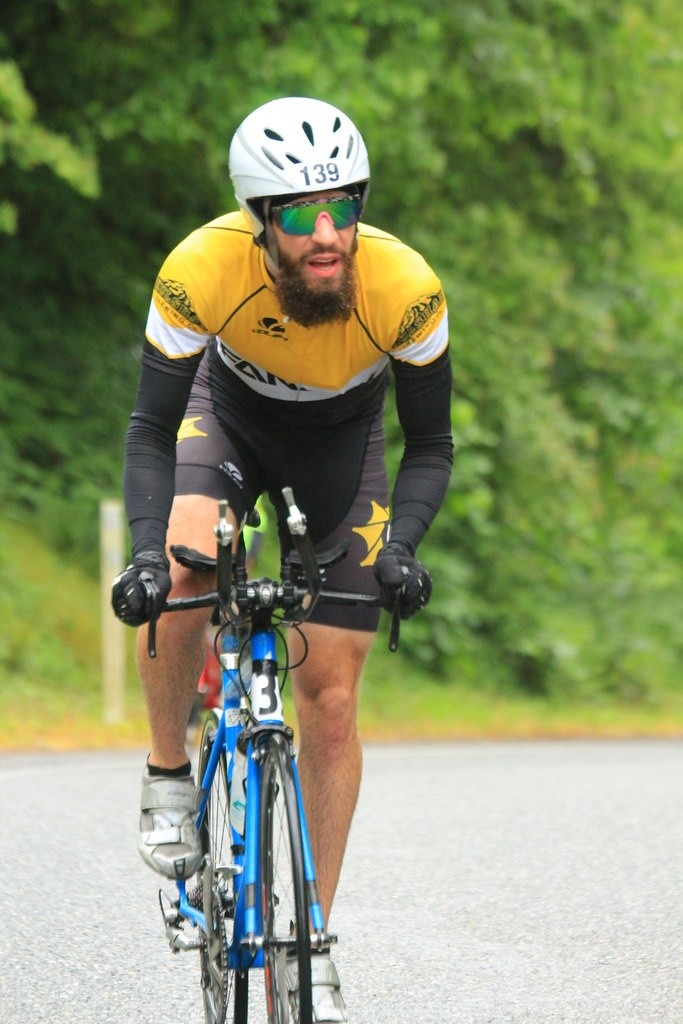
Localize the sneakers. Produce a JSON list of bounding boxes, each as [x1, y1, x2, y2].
[[275, 951, 349, 1024], [136, 758, 202, 879]]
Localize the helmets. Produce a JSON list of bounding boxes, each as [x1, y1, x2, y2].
[[228, 97, 373, 238]]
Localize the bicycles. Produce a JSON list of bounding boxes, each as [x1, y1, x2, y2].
[[136, 486, 408, 1024]]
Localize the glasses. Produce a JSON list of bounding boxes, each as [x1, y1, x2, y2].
[[270, 194, 361, 236]]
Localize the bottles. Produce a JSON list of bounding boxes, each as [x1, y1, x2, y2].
[[230, 729, 253, 835]]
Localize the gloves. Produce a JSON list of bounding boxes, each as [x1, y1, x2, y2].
[[371, 541, 432, 620], [110, 557, 172, 627]]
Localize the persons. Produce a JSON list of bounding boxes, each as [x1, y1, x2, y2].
[[108, 98, 454, 1023]]
